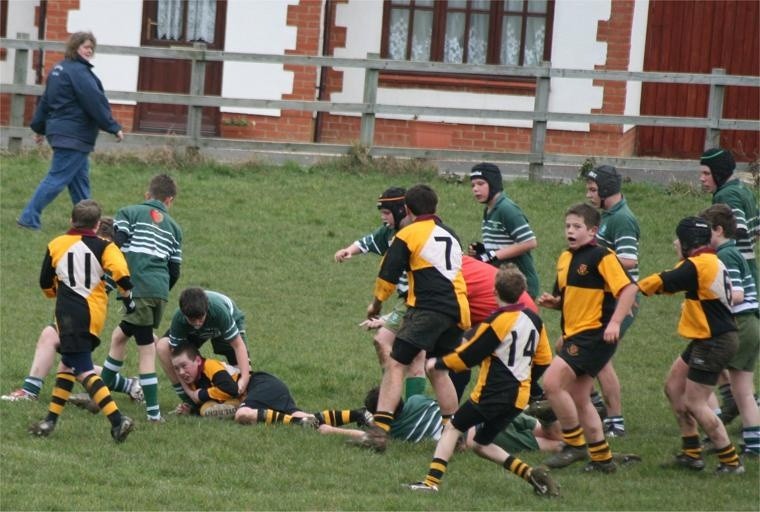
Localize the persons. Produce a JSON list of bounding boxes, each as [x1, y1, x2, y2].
[[317, 146, 760, 498], [68, 173, 182, 424], [16, 32, 125, 233], [155, 287, 252, 417], [169, 342, 374, 429], [0, 217, 147, 406], [28, 198, 135, 443]]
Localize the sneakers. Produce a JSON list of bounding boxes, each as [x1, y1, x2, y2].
[[754, 394, 760, 404], [68, 392, 99, 414], [593, 401, 608, 418], [529, 465, 561, 496], [401, 481, 439, 491], [298, 417, 320, 430], [1, 389, 39, 403], [362, 407, 374, 427], [738, 449, 759, 458], [148, 416, 167, 423], [168, 404, 196, 416], [714, 459, 745, 476], [128, 376, 144, 404], [579, 459, 617, 473], [603, 425, 625, 438], [345, 428, 387, 452], [717, 402, 740, 424], [110, 416, 135, 443], [700, 436, 717, 453], [541, 445, 588, 468], [660, 451, 705, 470], [28, 419, 54, 437]]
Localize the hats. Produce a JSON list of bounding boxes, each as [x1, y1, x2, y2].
[[377, 186, 407, 221], [587, 165, 622, 199], [469, 162, 503, 192], [676, 218, 712, 259], [699, 149, 736, 187]]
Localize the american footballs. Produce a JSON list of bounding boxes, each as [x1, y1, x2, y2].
[[204, 404, 237, 421]]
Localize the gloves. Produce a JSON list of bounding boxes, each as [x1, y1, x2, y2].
[[116, 291, 135, 315], [476, 251, 497, 263], [472, 241, 485, 256]]
